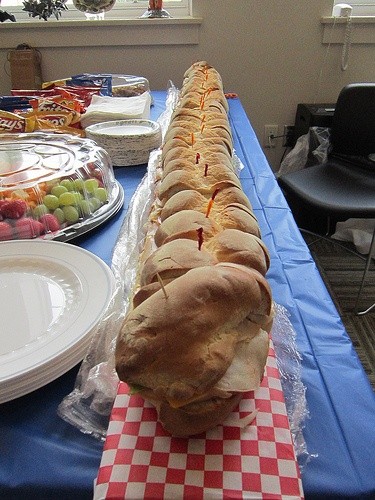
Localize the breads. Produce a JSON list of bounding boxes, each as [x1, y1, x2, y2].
[[114, 61, 272, 438]]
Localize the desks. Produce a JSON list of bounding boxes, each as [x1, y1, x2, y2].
[[0, 89, 375, 500]]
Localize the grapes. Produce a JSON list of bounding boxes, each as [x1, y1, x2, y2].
[[32, 178, 108, 224]]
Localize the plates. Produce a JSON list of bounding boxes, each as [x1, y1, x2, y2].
[[84, 117, 164, 167], [0, 240, 120, 407]]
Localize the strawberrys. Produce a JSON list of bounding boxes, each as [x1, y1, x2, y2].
[[0, 200, 58, 241]]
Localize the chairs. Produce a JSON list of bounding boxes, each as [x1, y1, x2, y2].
[[272, 82, 375, 319]]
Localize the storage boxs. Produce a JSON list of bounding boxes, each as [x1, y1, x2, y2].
[[6, 49, 45, 91]]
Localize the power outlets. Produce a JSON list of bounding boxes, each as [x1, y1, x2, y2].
[[262, 124, 279, 149]]
